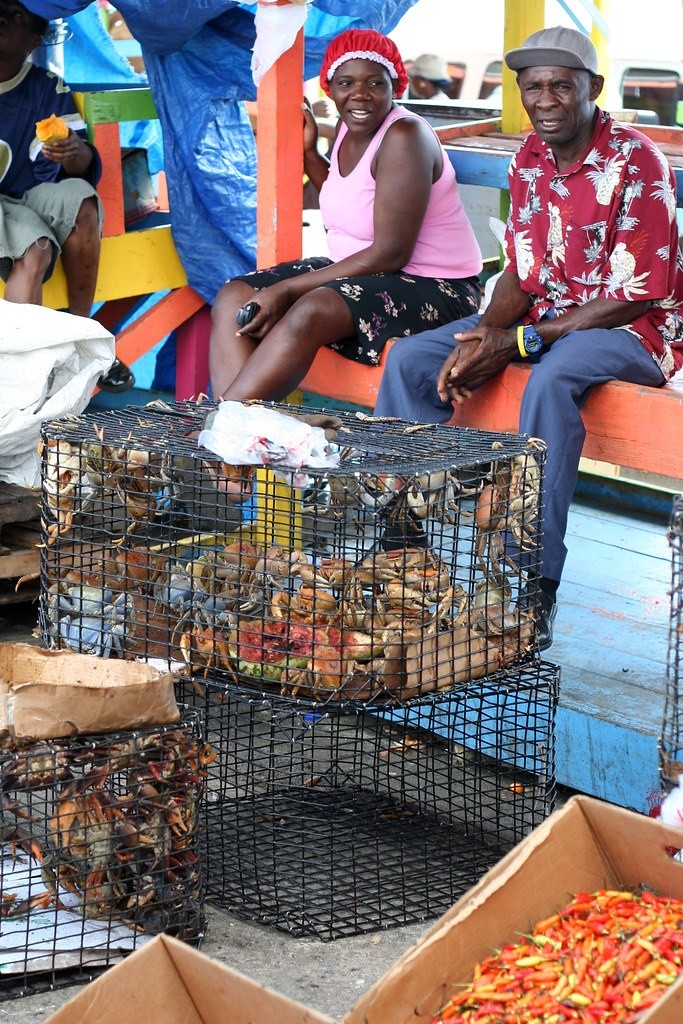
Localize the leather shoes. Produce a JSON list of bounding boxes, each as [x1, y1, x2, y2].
[[514, 587, 558, 651], [380, 507, 427, 552], [98, 358, 135, 392]]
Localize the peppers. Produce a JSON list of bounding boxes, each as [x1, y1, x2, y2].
[[428, 891, 683, 1024]]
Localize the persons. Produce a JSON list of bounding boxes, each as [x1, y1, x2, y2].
[[0, 0, 136, 394], [331, 27, 683, 653], [207, 29, 483, 503], [407, 54, 453, 99]]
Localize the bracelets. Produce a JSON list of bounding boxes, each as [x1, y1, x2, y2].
[[517, 325, 530, 358]]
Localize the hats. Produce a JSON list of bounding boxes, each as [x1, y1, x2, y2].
[[320, 28, 411, 101], [407, 54, 452, 84], [503, 26, 598, 76]]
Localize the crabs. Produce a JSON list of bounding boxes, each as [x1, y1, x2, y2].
[[1, 729, 218, 938], [15, 393, 547, 702]]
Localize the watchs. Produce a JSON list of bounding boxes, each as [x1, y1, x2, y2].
[[523, 325, 544, 354]]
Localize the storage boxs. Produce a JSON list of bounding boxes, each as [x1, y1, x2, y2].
[[332, 792, 683, 1024], [40, 931, 342, 1024]]
